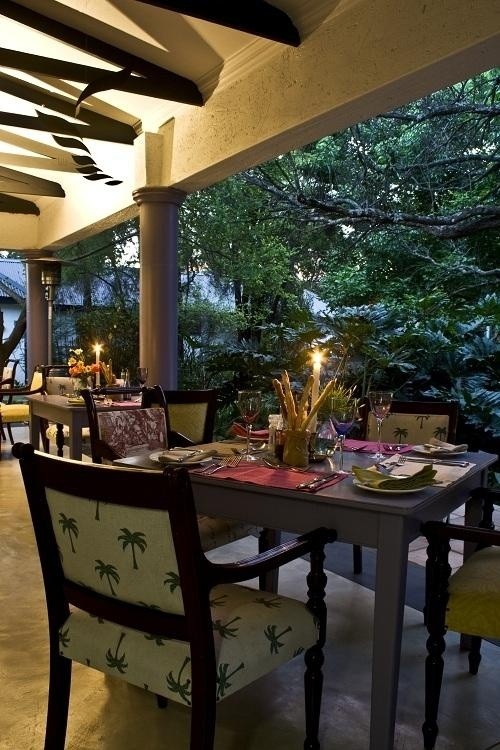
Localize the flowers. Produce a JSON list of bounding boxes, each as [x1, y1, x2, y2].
[[66, 359, 102, 378]]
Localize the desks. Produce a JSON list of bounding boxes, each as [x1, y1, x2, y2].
[[28, 395, 141, 462]]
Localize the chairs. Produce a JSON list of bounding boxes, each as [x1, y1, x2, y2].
[[140, 387, 221, 447], [82, 385, 197, 464], [2, 360, 70, 445], [12, 443, 338, 749], [421, 488, 499, 749], [353, 400, 459, 575]]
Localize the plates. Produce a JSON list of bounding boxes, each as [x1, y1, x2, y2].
[[149, 450, 213, 466], [353, 475, 430, 493], [412, 444, 467, 458], [66, 397, 86, 407]]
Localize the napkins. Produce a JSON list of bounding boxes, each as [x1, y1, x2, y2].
[[352, 464, 444, 491], [340, 439, 415, 455], [232, 422, 276, 439], [188, 460, 345, 494], [424, 439, 471, 455], [160, 446, 216, 464], [187, 440, 274, 458]]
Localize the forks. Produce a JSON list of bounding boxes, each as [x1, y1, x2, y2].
[[385, 455, 408, 472], [194, 455, 243, 476]]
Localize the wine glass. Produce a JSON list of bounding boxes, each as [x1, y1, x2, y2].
[[238, 390, 262, 461], [134, 368, 149, 402], [331, 396, 356, 475], [368, 390, 393, 459]]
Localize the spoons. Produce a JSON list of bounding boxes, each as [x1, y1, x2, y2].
[[263, 458, 304, 470]]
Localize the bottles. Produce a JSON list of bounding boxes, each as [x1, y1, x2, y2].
[[123, 372, 131, 400]]
